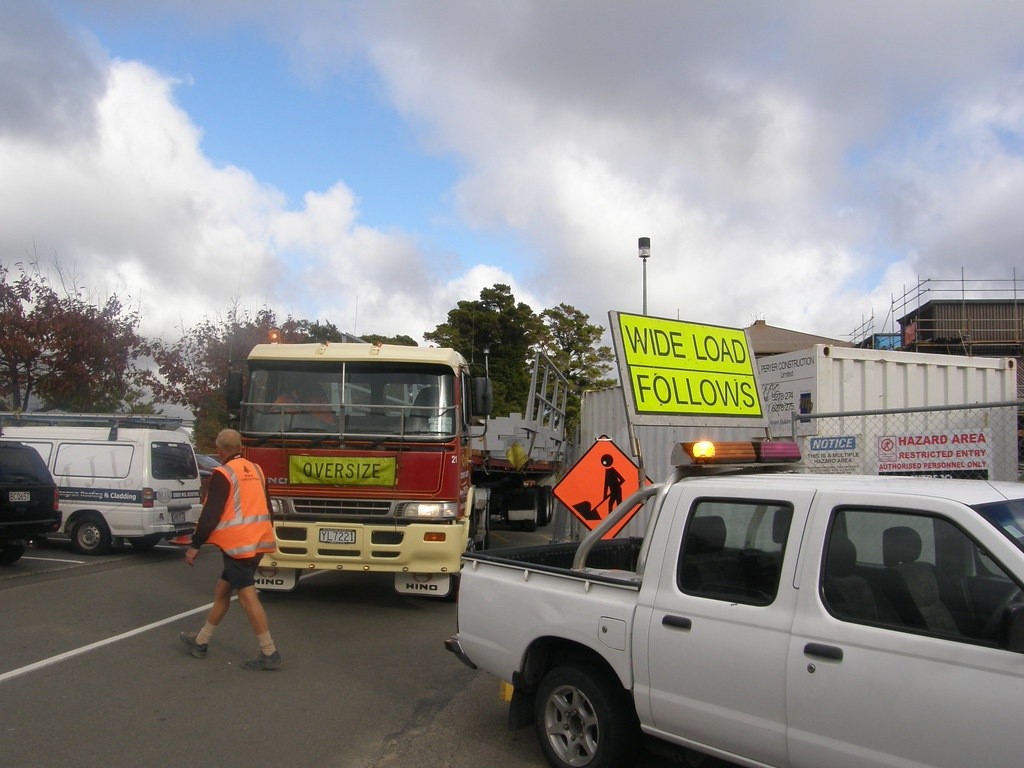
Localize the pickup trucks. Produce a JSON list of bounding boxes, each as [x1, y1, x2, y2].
[[445, 441, 1024, 768]]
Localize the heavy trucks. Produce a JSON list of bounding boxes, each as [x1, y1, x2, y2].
[[227, 330, 568, 604]]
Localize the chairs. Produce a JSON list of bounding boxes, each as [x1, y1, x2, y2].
[[879, 526, 962, 634], [408, 387, 438, 431], [826, 537, 903, 627], [683, 516, 736, 595], [772, 510, 793, 558]]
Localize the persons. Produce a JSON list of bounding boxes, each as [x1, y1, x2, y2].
[[178, 429, 281, 668], [272, 377, 334, 425]]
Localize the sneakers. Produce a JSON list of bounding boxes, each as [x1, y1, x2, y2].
[[242, 651, 282, 672], [178, 631, 208, 659]]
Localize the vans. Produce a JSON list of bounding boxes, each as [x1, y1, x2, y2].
[[0, 411, 204, 557]]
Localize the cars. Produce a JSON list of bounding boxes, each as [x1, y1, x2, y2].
[[195, 454, 222, 478], [0, 440, 62, 568]]
